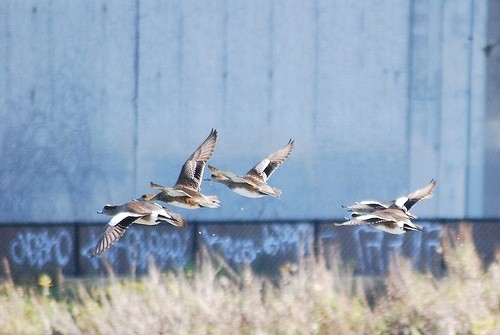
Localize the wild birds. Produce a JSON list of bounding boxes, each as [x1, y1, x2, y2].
[[131, 128, 221, 210], [202, 138, 295, 199], [89, 192, 188, 258], [332, 178, 438, 235]]
